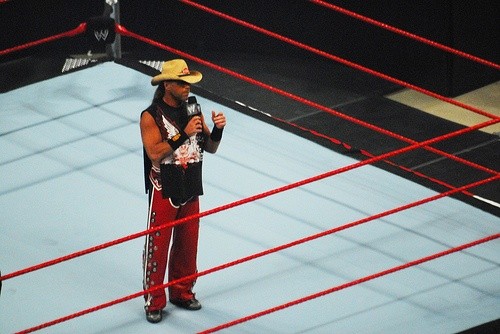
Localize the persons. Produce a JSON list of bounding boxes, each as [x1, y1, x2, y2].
[[140, 59, 226, 323]]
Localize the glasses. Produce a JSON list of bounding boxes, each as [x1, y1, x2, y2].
[[177, 80, 186, 85]]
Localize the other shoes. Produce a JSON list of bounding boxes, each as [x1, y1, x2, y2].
[[170, 298, 201, 310], [146, 310, 162, 323]]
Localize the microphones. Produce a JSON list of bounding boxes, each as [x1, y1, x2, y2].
[[186, 96, 203, 137]]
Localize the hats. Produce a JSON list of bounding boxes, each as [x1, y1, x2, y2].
[[151, 59, 203, 86]]
